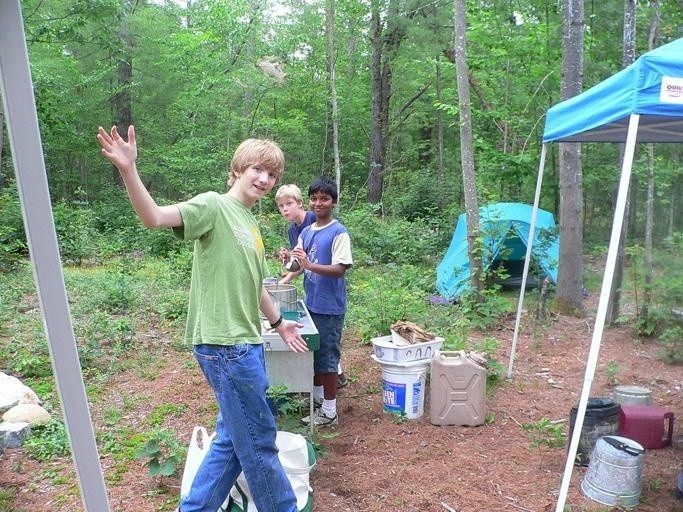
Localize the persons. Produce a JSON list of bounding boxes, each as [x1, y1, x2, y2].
[[96, 125, 309, 512], [270, 182, 348, 390], [279, 177, 354, 429]]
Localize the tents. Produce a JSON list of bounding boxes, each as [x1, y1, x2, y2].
[[436, 199, 589, 302]]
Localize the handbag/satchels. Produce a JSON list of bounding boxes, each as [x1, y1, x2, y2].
[[229, 431, 317, 512]]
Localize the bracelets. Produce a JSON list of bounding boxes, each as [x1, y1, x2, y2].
[[270, 315, 283, 327]]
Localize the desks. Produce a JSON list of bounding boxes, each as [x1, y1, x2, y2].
[[255, 298, 320, 437]]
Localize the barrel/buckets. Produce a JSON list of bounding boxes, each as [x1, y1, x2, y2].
[[566, 398, 621, 467], [430, 349, 487, 427], [379, 364, 427, 419], [619, 405, 674, 449], [579, 435, 644, 508], [615, 387, 654, 409]]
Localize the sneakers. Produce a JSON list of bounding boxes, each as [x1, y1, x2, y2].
[[306, 413, 338, 429], [300, 409, 321, 427], [336, 373, 347, 390], [302, 397, 324, 412]]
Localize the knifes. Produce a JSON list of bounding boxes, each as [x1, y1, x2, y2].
[[601, 436, 644, 455]]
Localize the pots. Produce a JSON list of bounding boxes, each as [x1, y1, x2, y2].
[[262, 284, 296, 316]]
